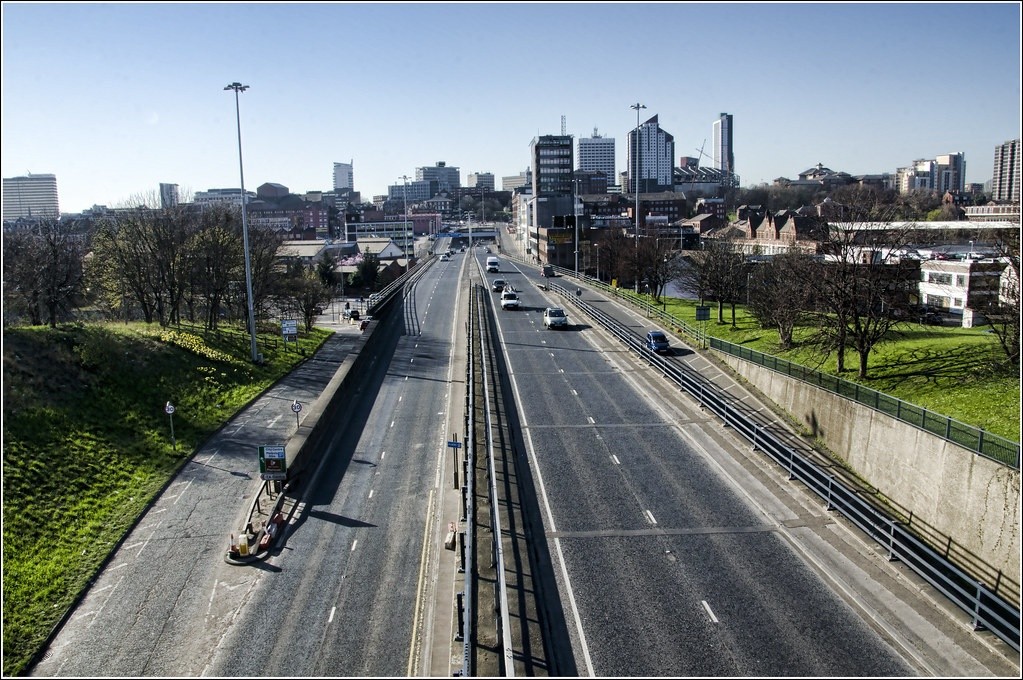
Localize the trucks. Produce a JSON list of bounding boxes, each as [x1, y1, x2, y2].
[[485, 257, 500, 274]]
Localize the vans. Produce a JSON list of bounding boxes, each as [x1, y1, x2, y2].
[[501, 291, 519, 310]]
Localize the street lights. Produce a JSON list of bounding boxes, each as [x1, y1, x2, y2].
[[398, 175, 412, 272], [630, 103, 647, 295], [223, 82, 263, 364], [572, 178, 582, 278]]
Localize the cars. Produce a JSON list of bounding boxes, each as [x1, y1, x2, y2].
[[343, 308, 373, 331], [646, 330, 671, 356], [543, 308, 569, 330], [475, 239, 493, 254], [311, 303, 328, 315], [492, 279, 506, 293], [440, 247, 465, 262]]
